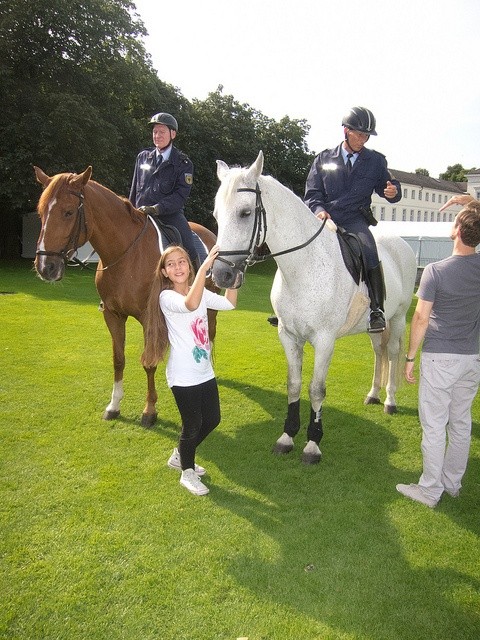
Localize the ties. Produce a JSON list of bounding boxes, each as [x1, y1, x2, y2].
[[346, 154, 353, 177], [156, 156, 162, 171]]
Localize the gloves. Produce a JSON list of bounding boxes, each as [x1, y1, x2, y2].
[[138, 206, 158, 217]]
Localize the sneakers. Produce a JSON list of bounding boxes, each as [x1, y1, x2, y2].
[[167, 447, 205, 475], [396, 483, 437, 508], [179, 467, 209, 495], [441, 476, 458, 498]]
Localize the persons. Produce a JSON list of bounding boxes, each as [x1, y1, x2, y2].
[[396, 193, 480, 508], [100, 113, 200, 309], [267, 107, 402, 333], [141, 246, 242, 496]]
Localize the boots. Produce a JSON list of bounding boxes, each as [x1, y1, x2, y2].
[[365, 260, 385, 328]]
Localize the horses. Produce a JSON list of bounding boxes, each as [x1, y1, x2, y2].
[[33, 165, 222, 427], [211, 149, 418, 465]]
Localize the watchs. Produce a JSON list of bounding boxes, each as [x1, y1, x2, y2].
[[406, 354, 416, 362]]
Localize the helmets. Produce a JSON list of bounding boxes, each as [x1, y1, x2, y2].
[[147, 112, 179, 150], [341, 106, 377, 153]]
